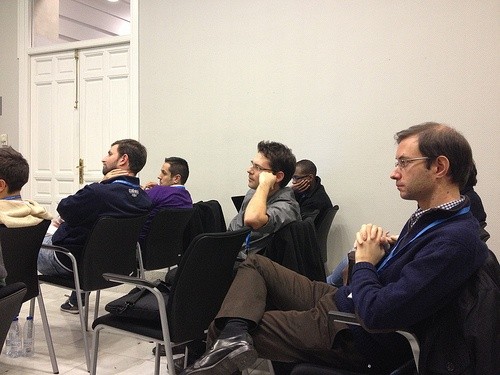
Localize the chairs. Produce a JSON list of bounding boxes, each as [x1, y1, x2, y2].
[[0, 200, 500, 375]]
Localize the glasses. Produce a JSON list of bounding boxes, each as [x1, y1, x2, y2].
[[249, 162, 272, 171], [394, 157, 430, 167], [292, 174, 310, 180]]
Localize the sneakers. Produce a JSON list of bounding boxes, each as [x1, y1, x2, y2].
[[61, 300, 79, 313]]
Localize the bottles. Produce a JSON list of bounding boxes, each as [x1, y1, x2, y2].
[[23, 316, 35, 357], [10, 318, 22, 357]]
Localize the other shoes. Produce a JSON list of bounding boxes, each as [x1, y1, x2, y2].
[[167, 356, 192, 372], [152, 342, 185, 356]]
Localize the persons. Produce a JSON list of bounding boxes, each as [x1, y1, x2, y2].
[[37, 139, 147, 285], [180, 121, 490, 375], [0, 146, 53, 228], [61, 157, 193, 314], [291, 160, 333, 230], [152, 141, 303, 374]]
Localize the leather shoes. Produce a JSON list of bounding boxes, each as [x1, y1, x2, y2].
[[179, 332, 257, 375]]
